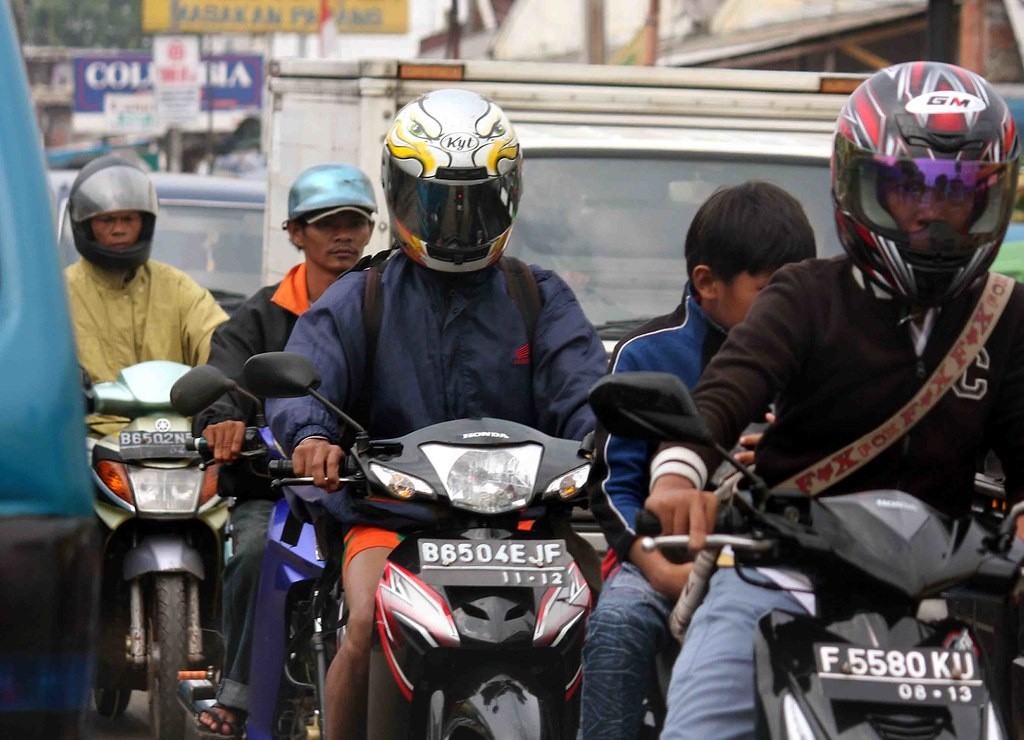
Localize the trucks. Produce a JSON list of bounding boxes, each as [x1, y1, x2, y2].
[[259, 54, 879, 390]]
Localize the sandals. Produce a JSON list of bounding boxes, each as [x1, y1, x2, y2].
[[194, 699, 248, 738]]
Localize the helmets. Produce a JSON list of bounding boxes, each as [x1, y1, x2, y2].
[[829, 59, 1021, 304], [288, 164, 378, 225], [67, 154, 158, 272], [379, 90, 526, 280]]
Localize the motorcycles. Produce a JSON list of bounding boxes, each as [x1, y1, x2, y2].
[[77, 357, 1024, 740]]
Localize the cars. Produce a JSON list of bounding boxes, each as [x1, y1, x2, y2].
[[51, 163, 266, 327]]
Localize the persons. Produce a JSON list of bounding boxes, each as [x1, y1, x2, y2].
[[193, 61, 1024, 740], [52, 158, 233, 670]]
